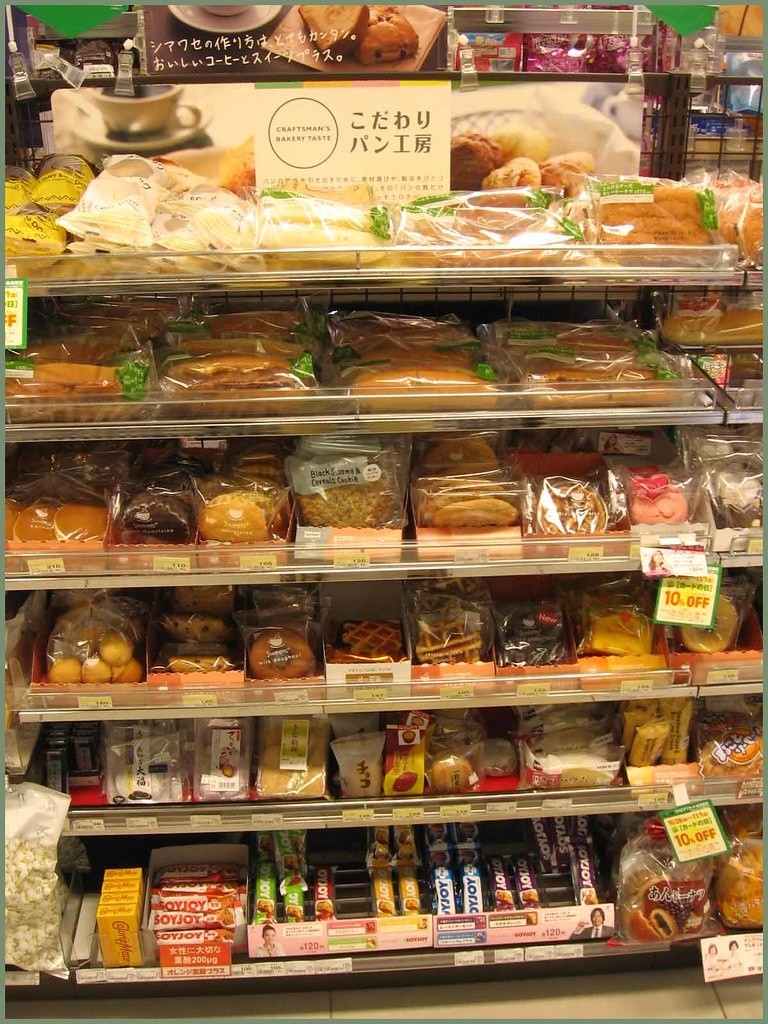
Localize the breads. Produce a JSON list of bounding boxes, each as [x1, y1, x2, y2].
[[298, 4, 419, 65], [5, 159, 765, 942], [451, 128, 597, 189]]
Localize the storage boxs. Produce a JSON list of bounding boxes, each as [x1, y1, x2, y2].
[[29, 453, 766, 969], [455, 32, 654, 73]]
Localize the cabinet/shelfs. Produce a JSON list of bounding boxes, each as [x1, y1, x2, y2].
[[662, 266, 763, 956], [6, 246, 768, 986], [6, 723, 86, 987]]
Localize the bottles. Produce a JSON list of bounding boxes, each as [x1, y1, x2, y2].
[[726, 118, 747, 151]]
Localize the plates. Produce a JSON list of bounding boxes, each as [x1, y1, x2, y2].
[[71, 101, 214, 156], [166, 5, 284, 33]]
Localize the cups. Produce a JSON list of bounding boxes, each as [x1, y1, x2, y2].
[[93, 85, 201, 137]]
